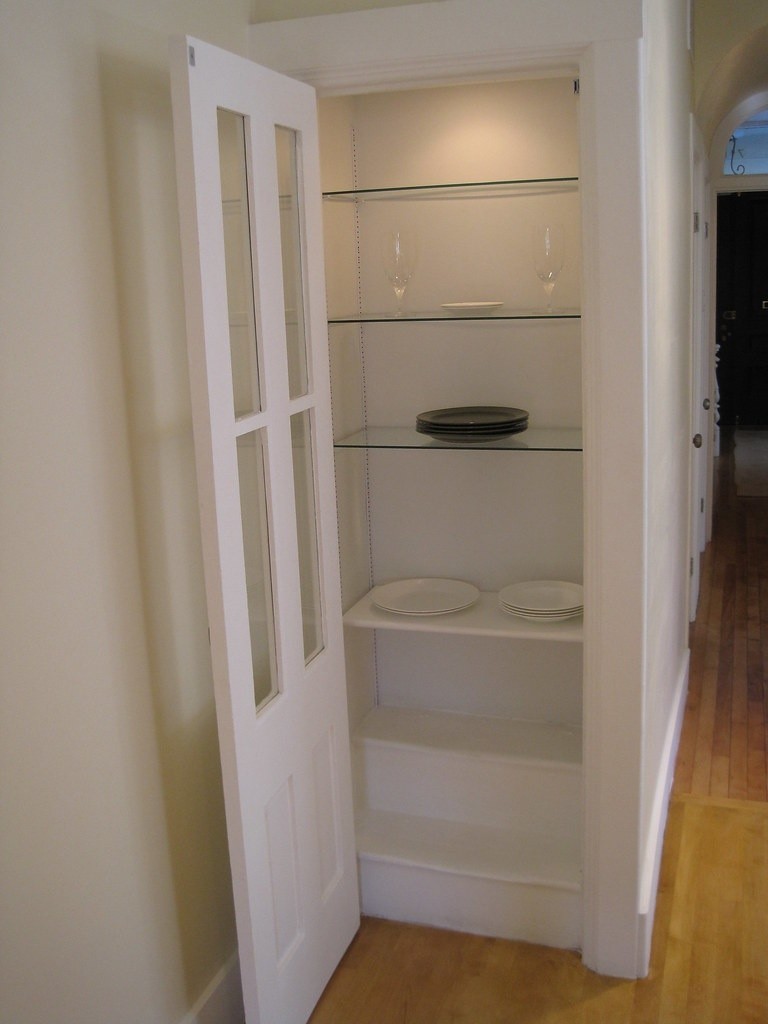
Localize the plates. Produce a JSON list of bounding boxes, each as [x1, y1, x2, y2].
[[500, 580, 583, 623], [440, 302, 504, 317], [373, 578, 481, 616], [416, 406, 529, 444]]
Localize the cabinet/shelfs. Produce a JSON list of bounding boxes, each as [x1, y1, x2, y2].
[[324, 177, 582, 771]]
